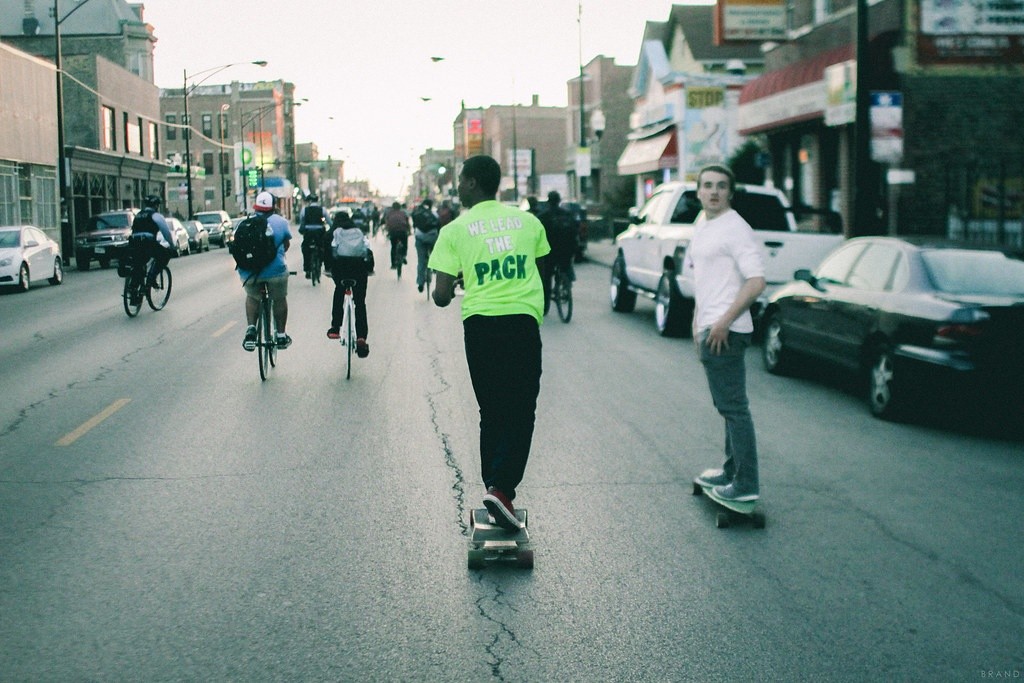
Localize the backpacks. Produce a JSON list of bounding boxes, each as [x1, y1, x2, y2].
[[331, 226, 370, 259], [225, 212, 284, 273]]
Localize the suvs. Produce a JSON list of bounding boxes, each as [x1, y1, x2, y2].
[[519, 199, 588, 261]]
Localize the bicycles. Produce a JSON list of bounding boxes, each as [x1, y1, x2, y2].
[[122, 238, 576, 384]]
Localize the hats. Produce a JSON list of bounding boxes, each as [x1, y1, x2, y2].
[[303, 193, 319, 201], [252, 191, 276, 212]]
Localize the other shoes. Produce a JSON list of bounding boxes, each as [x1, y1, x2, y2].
[[390, 263, 396, 270], [146, 278, 160, 289], [402, 258, 408, 265], [129, 297, 139, 306], [418, 281, 424, 293], [305, 271, 312, 278]]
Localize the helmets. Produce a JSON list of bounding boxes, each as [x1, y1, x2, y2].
[[144, 195, 162, 204]]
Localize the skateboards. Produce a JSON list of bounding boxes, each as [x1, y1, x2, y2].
[[464, 506, 539, 571], [684, 472, 766, 534]]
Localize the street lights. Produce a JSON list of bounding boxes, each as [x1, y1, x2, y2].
[[241, 102, 301, 215], [182, 61, 266, 221]]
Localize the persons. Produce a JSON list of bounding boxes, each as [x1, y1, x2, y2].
[[382, 201, 414, 270], [297, 192, 327, 285], [126, 194, 174, 307], [675, 164, 773, 506], [415, 198, 440, 293], [323, 207, 376, 359], [608, 128, 677, 177], [401, 202, 413, 235], [538, 191, 577, 315], [437, 190, 541, 230], [427, 156, 556, 540], [230, 190, 292, 353], [371, 206, 379, 238]]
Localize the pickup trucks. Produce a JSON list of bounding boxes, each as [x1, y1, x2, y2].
[[608, 181, 845, 340]]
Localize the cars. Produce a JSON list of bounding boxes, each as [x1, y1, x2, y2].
[[0, 208, 255, 292], [760, 235, 1024, 418]]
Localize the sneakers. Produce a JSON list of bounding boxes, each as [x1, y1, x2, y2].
[[242, 327, 257, 352], [356, 337, 369, 358], [483, 485, 522, 533], [277, 334, 292, 350], [696, 471, 733, 488], [712, 484, 761, 501], [327, 326, 340, 339]]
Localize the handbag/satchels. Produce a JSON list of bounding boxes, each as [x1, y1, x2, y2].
[[118, 247, 137, 278], [415, 227, 439, 246]]
